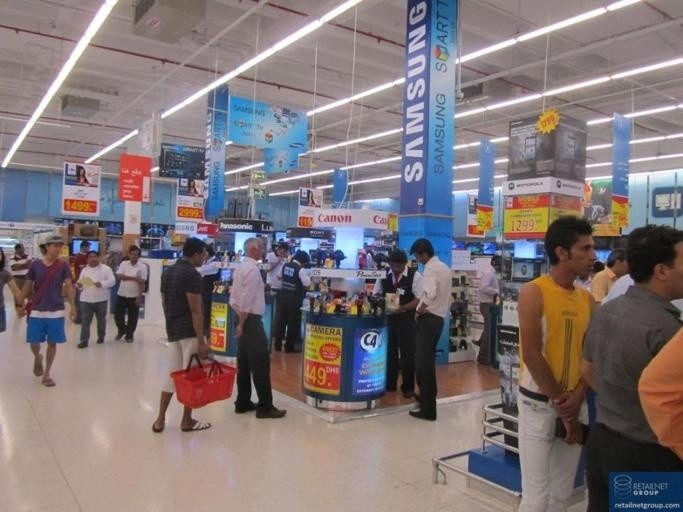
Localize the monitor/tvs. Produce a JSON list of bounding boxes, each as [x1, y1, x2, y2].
[[71, 239, 101, 256], [139, 237, 162, 251]]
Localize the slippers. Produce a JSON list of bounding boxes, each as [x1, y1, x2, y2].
[[182, 419, 211, 431], [153, 421, 164, 432]]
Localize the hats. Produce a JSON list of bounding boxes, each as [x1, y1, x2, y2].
[[38, 232, 64, 247], [80, 240, 91, 246]]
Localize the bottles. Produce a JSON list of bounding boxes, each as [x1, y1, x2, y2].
[[359, 249, 367, 269]]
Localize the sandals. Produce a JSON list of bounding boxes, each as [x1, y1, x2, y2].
[[42, 378, 55, 386], [33, 353, 44, 376]]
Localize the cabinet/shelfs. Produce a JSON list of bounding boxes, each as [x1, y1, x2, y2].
[[449, 263, 479, 364]]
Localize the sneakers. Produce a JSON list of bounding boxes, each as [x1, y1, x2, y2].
[[97, 337, 104, 343], [117, 325, 133, 343], [235, 403, 286, 418], [285, 348, 302, 353], [78, 342, 87, 348], [474, 341, 480, 346]]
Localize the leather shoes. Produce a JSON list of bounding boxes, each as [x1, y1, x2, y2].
[[409, 410, 436, 420], [386, 387, 421, 403]]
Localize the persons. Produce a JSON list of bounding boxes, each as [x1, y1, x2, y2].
[[273, 251, 312, 352], [265, 241, 293, 335], [518, 216, 683, 511], [152, 236, 213, 432], [471, 255, 503, 369], [78, 166, 90, 186], [196, 241, 222, 338], [409, 239, 453, 421], [228, 237, 287, 417], [1, 233, 147, 385], [374, 249, 423, 398], [189, 180, 199, 196]]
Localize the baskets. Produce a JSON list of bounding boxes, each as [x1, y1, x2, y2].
[[170, 353, 240, 408]]
